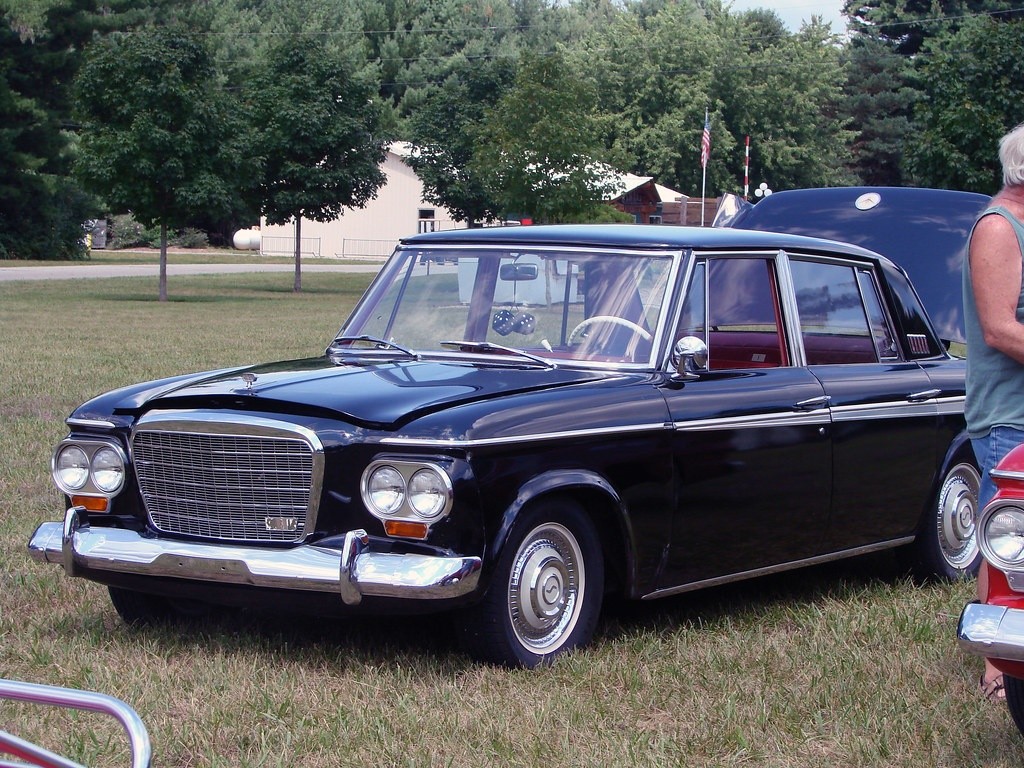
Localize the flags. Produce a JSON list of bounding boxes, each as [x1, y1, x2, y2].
[[700, 113, 711, 167]]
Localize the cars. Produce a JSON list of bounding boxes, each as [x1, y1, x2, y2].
[[27, 186, 994, 668]]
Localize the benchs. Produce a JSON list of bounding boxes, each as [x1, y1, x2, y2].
[[634, 331, 887, 369]]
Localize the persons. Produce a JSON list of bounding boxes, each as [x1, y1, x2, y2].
[[960, 120, 1023, 703]]
